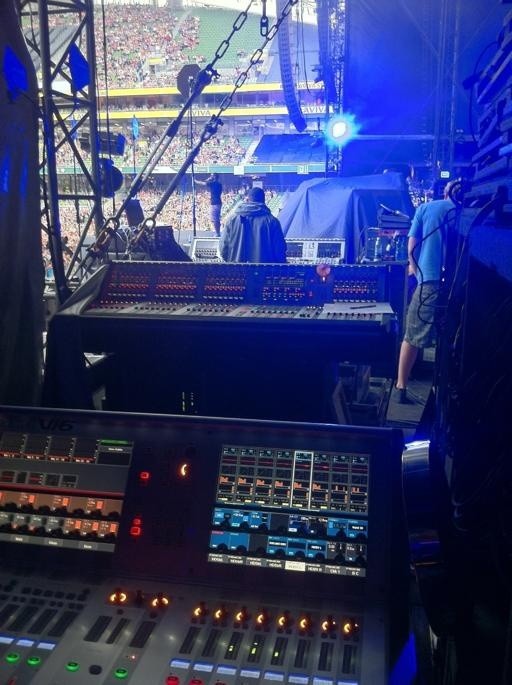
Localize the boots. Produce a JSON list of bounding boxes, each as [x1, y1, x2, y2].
[[389, 380, 406, 403]]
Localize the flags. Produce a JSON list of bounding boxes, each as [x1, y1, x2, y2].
[[71, 117, 77, 140], [133, 116, 139, 137]]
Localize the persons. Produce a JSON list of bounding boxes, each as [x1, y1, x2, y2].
[[219, 187, 287, 264], [194, 173, 223, 236], [40, 189, 277, 268], [38, 123, 246, 166], [48, 5, 254, 90], [390, 179, 471, 403]]
